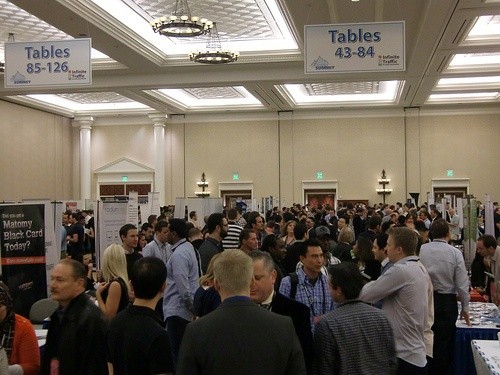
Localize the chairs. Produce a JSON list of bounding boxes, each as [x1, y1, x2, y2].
[[29, 298, 61, 324]]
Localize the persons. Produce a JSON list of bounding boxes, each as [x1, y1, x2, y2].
[[0, 200, 500, 375]]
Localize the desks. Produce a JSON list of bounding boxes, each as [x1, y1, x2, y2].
[[471, 340, 500, 375], [456, 286, 487, 302], [454, 301, 500, 375], [31, 324, 49, 352]]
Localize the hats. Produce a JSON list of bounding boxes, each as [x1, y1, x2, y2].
[[413, 221, 428, 231], [315, 226, 330, 237]]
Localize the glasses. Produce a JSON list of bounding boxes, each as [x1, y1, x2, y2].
[[274, 234, 280, 240], [220, 224, 228, 226]]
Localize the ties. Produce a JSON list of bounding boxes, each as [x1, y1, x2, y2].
[[260, 303, 271, 311]]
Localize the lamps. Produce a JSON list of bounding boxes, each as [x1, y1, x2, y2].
[[195, 172, 211, 198], [188, 21, 241, 63], [150, 0, 214, 37], [376, 169, 392, 204]]
